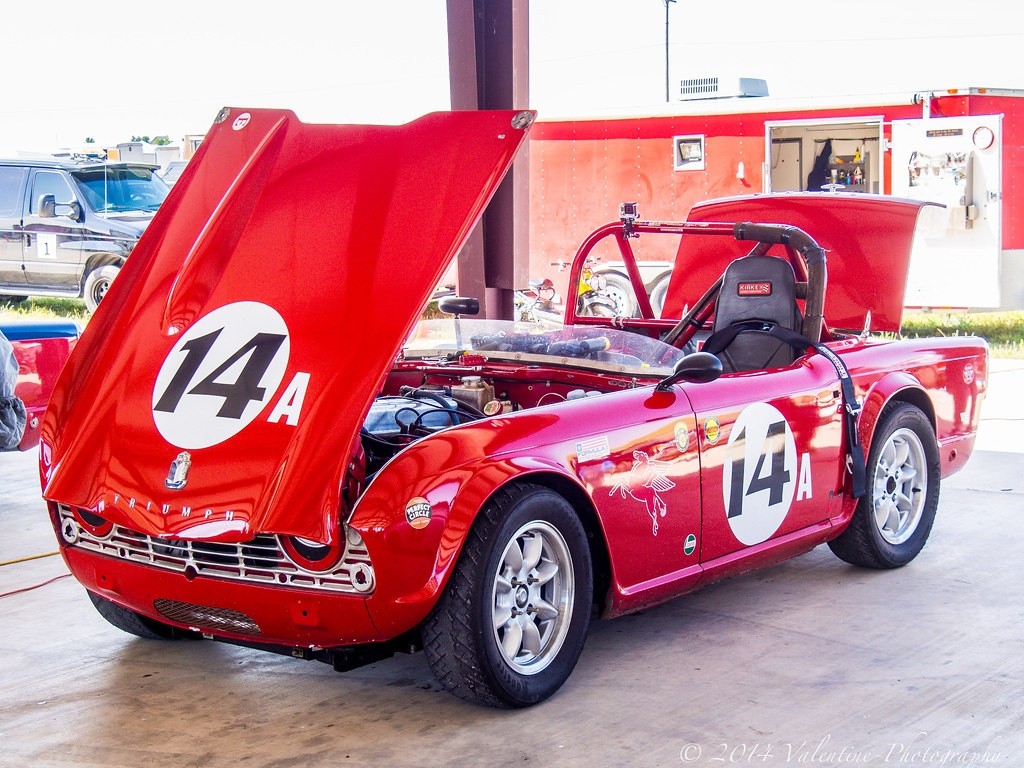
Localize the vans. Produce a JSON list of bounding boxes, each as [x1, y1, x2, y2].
[[0, 148, 173, 318]]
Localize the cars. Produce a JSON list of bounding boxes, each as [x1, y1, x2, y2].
[[36, 107, 988, 711]]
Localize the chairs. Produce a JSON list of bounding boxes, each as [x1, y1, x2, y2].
[[704, 256, 804, 366]]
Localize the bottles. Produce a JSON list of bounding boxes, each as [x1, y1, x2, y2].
[[831, 166, 863, 185], [855, 148, 859, 161]]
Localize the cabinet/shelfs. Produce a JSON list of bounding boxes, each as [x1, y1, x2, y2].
[[816, 152, 870, 194]]
